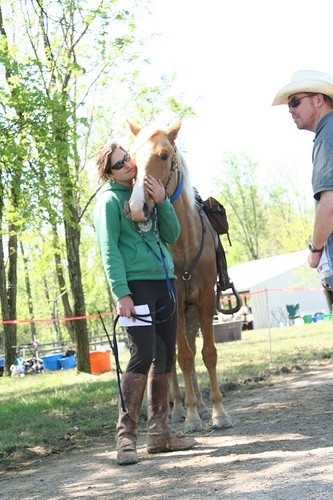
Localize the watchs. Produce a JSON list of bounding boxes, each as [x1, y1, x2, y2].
[[309, 243, 324, 253]]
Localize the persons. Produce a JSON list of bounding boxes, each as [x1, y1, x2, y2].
[[272, 71, 333, 272], [92, 142, 196, 464]]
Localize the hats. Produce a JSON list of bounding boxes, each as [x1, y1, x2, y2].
[[272, 70, 333, 106]]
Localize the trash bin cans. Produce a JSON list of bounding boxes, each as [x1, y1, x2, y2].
[[213, 321, 242, 343], [57, 355, 76, 370], [312, 316, 318, 323], [41, 353, 62, 371], [89, 350, 111, 374], [302, 314, 312, 324]]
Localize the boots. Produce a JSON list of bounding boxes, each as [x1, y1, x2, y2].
[[145, 371, 195, 453], [116, 372, 147, 464]]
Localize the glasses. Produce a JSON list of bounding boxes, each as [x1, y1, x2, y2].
[[107, 153, 130, 170], [289, 95, 314, 107]]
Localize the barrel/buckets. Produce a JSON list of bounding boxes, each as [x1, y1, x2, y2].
[[58, 354, 77, 370], [40, 353, 62, 371], [90, 350, 112, 374], [303, 315, 311, 323]]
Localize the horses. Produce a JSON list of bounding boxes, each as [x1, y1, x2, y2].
[[124, 114, 235, 432]]
